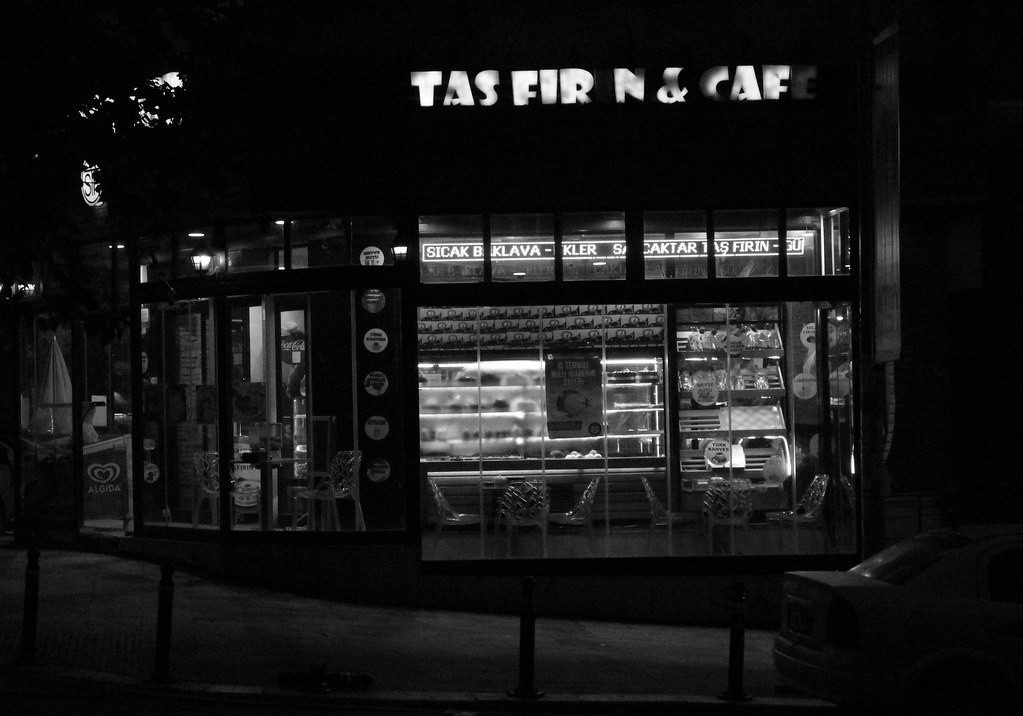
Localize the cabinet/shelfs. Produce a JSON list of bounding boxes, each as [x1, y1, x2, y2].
[[415, 346, 667, 526], [676, 320, 791, 514]]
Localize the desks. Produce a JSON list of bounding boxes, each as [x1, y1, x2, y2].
[[470, 475, 562, 531], [230, 458, 309, 531], [678, 485, 761, 554]]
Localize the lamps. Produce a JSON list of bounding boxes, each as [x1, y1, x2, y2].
[[390, 233, 409, 279], [188, 237, 215, 287]]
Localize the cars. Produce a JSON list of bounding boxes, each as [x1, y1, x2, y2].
[[772, 523, 1023, 716]]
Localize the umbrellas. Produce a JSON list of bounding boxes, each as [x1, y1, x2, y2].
[[25, 339, 98, 444]]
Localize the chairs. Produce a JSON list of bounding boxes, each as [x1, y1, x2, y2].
[[547, 475, 598, 535], [638, 474, 699, 538], [699, 481, 754, 555], [291, 450, 368, 532], [763, 474, 832, 554], [191, 451, 263, 529], [494, 478, 550, 536], [425, 475, 486, 535]]
